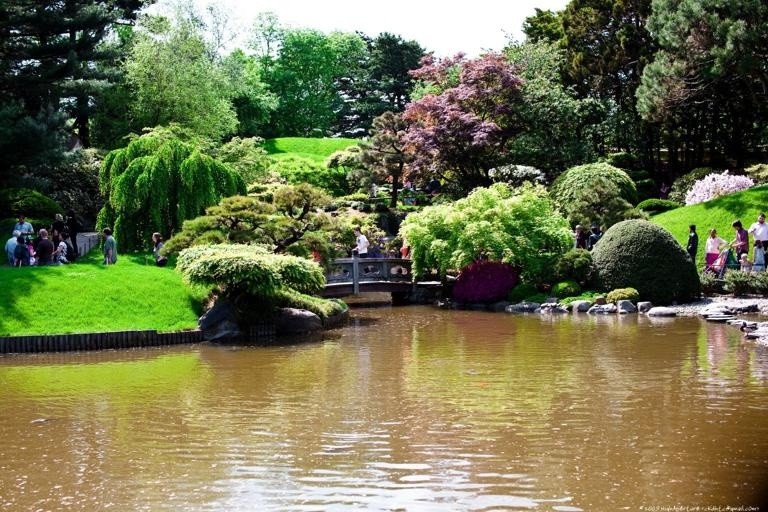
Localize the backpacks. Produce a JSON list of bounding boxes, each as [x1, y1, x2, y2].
[[58, 240, 76, 261]]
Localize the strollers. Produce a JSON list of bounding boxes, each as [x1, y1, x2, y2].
[[704, 244, 733, 279]]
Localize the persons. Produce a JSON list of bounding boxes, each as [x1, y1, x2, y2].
[[736, 251, 752, 276], [151, 230, 169, 267], [3, 211, 77, 268], [752, 238, 767, 272], [704, 228, 728, 273], [747, 212, 767, 273], [350, 225, 371, 259], [100, 227, 118, 265], [366, 174, 441, 206], [400, 235, 412, 275], [659, 181, 669, 200], [728, 219, 749, 266], [573, 224, 585, 249], [587, 221, 604, 252], [685, 223, 698, 264]]
